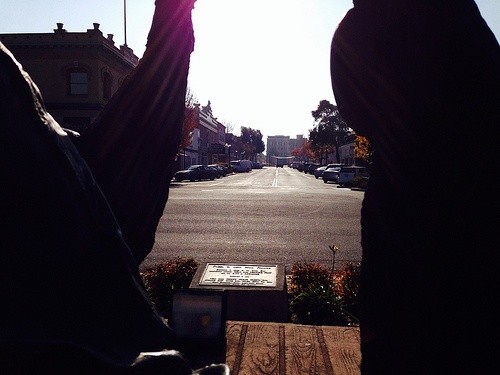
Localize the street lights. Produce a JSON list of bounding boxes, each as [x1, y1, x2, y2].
[[224, 142, 232, 169]]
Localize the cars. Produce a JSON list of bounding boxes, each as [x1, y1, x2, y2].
[[172, 159, 265, 183], [288, 160, 371, 189]]
